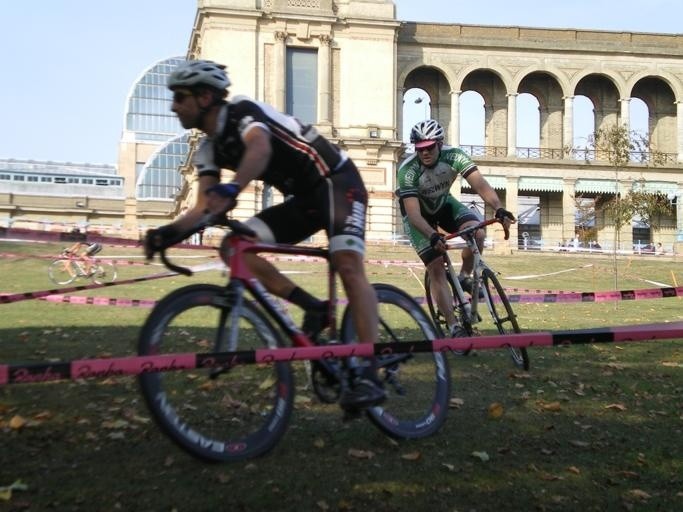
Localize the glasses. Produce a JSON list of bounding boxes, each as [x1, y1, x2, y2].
[[416, 145, 436, 152], [172, 91, 198, 101]]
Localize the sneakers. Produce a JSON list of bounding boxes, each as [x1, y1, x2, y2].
[[340, 367, 388, 411], [457, 275, 484, 299], [301, 300, 333, 341], [449, 326, 472, 356]]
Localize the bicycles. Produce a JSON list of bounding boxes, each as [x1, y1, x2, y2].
[[49, 247, 118, 287]]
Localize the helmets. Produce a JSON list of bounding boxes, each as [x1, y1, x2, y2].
[[410, 119, 444, 147], [69, 227, 82, 234], [168, 59, 231, 94]]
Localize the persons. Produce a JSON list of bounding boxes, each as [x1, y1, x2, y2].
[[144, 60, 387, 409], [64, 229, 102, 276], [394, 119, 517, 352]]
